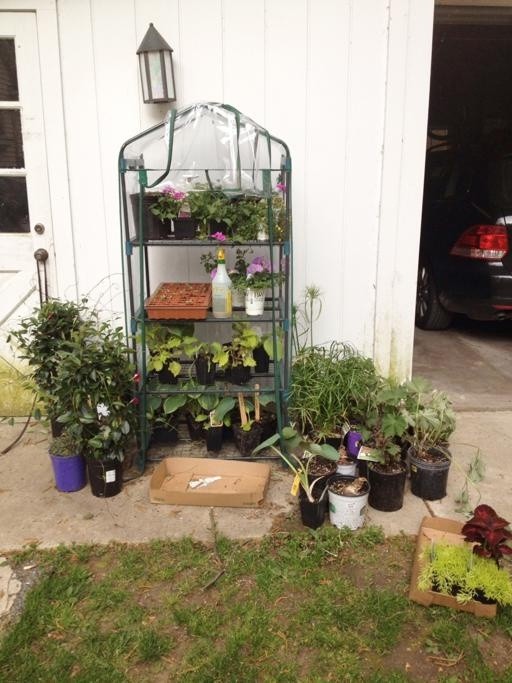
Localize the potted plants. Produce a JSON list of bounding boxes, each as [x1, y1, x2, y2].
[[250, 427, 339, 531], [1, 263, 140, 497], [417, 544, 510, 609], [183, 329, 228, 386], [255, 330, 285, 374], [143, 281, 211, 320], [191, 183, 289, 240], [349, 375, 425, 513], [286, 282, 380, 453], [144, 391, 184, 446], [225, 321, 260, 385], [334, 444, 355, 475], [327, 474, 370, 531], [380, 374, 486, 514], [185, 392, 235, 455], [231, 383, 289, 457], [460, 503, 511, 564], [135, 323, 182, 384]]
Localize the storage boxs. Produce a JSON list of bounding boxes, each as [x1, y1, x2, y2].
[[149, 458, 271, 509], [405, 515, 504, 622]]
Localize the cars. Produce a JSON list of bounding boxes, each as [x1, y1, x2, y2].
[[415, 149, 512, 330]]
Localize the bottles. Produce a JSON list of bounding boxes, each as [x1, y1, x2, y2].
[[211, 260, 234, 318]]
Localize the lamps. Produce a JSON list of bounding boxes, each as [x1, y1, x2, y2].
[[136, 23, 177, 104]]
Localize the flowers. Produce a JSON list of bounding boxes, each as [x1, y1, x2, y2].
[[210, 231, 227, 240], [275, 183, 288, 195], [148, 186, 191, 218], [201, 248, 287, 287]]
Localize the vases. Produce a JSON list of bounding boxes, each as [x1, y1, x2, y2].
[[129, 192, 171, 241], [173, 218, 195, 240], [244, 287, 266, 316]]
